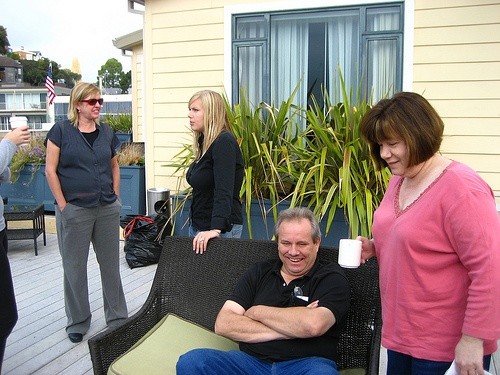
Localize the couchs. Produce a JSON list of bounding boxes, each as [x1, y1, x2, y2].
[[88, 235, 380, 375]]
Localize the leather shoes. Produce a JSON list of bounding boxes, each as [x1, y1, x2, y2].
[[68, 333, 83, 343]]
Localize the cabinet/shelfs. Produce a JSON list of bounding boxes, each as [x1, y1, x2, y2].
[[3, 205, 47, 257]]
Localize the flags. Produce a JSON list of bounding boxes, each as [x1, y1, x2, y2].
[[44, 65, 56, 105]]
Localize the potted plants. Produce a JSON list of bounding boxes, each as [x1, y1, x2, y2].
[[159, 65, 433, 248]]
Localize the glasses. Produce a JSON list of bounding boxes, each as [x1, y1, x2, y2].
[[293, 285, 302, 297], [80, 97, 104, 105]]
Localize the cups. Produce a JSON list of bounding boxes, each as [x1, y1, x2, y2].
[[9, 116, 29, 146], [338, 238, 362, 270]]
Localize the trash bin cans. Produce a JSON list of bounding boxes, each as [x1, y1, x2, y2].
[[146, 188, 170, 224]]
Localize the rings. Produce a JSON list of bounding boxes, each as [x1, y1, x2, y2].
[[200, 239, 204, 242]]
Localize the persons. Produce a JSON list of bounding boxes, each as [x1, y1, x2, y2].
[[184, 90, 244, 255], [176, 206, 353, 375], [352, 92, 499, 375], [45, 83, 128, 343], [0, 126, 33, 375]]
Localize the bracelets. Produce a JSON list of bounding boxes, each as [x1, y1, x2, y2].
[[210, 230, 221, 235]]
